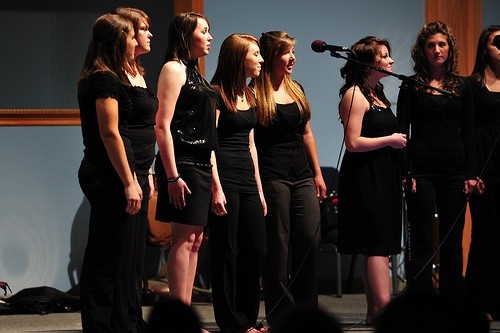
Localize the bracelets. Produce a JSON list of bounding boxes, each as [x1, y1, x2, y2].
[[166, 174, 180, 183]]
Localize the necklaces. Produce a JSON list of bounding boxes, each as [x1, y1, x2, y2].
[[236, 89, 245, 102]]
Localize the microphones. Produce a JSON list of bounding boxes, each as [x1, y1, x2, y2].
[[311, 40, 352, 53], [493, 35, 500, 50]]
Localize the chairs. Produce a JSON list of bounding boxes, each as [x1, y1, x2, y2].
[[314, 166, 343, 297]]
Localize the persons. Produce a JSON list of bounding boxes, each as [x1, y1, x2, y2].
[[154, 11, 217, 323], [249, 30, 327, 331], [338, 36, 408, 325], [77, 8, 500, 333]]
